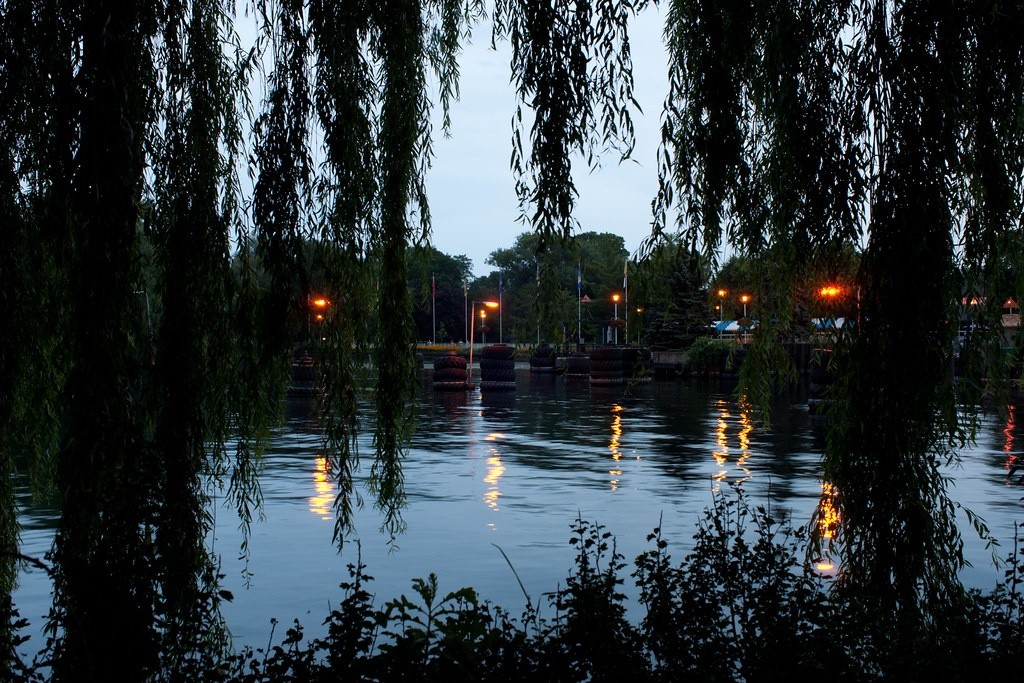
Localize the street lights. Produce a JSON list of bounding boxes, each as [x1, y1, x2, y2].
[[613, 295, 619, 346], [742, 296, 748, 343], [314, 297, 326, 343], [719, 290, 725, 340], [637, 308, 641, 344], [469, 300, 498, 396]]
